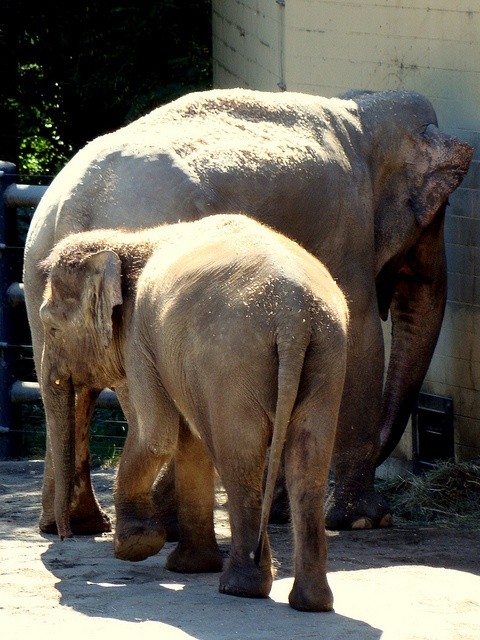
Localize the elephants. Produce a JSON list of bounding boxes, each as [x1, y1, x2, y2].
[[21, 87, 475, 532], [39, 214, 351, 612]]
